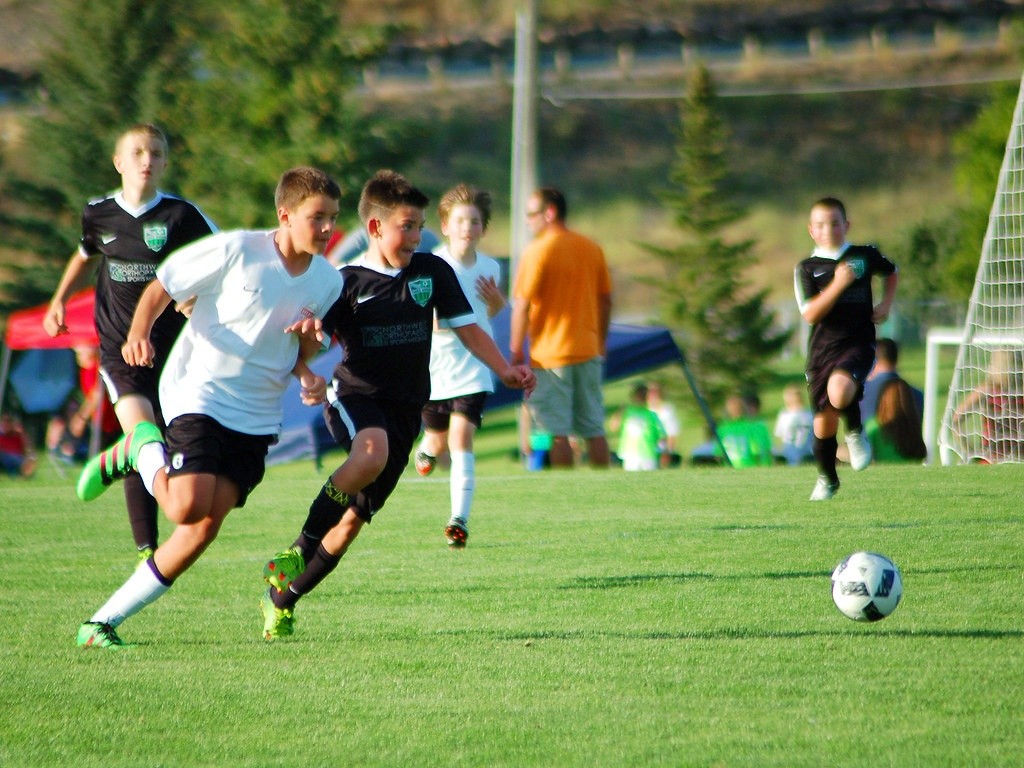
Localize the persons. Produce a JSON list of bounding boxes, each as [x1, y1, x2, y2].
[[412, 183, 505, 546], [329, 225, 439, 267], [520, 403, 553, 470], [774, 385, 813, 462], [510, 190, 610, 466], [837, 380, 926, 465], [793, 197, 899, 497], [43, 125, 216, 565], [953, 344, 1024, 465], [713, 393, 771, 468], [0, 350, 122, 476], [260, 169, 538, 638], [74, 168, 346, 650], [859, 338, 923, 424], [610, 380, 678, 469]]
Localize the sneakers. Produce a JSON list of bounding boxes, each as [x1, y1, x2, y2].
[[77, 421, 164, 502], [76, 622, 138, 650], [264, 545, 305, 593], [809, 477, 839, 500], [445, 518, 468, 549], [845, 426, 871, 471], [414, 433, 438, 477], [259, 589, 295, 642]]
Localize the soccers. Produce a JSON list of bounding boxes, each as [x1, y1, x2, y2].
[[830, 551, 903, 623]]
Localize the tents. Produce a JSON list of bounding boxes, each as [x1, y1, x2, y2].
[[0, 288, 104, 465], [267, 304, 738, 467]]
